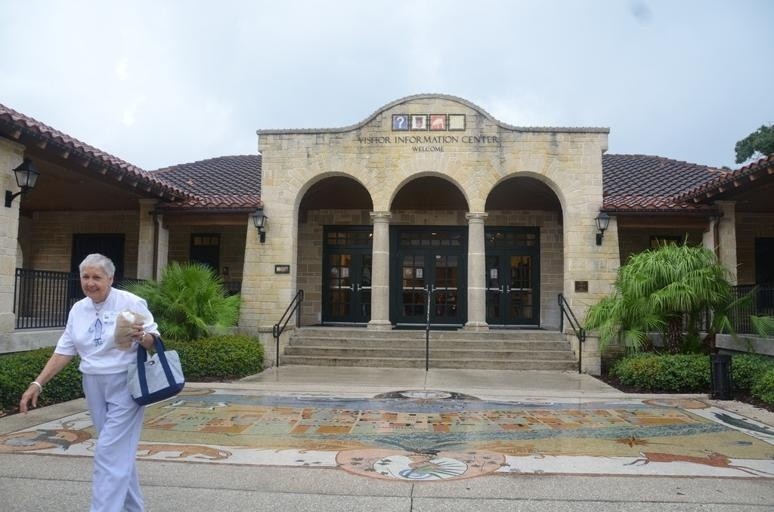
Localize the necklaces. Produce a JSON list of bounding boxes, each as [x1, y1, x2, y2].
[[91, 299, 105, 316]]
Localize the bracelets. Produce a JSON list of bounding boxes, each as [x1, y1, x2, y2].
[[137, 331, 146, 344], [31, 382, 42, 393]]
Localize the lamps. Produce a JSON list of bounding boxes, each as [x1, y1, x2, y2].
[[594, 207, 610, 245], [4, 156, 40, 208], [253, 203, 269, 242]]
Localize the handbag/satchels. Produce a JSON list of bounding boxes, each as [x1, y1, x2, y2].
[[127, 332, 186, 406]]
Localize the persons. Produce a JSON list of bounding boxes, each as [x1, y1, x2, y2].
[[17, 253, 186, 511]]
